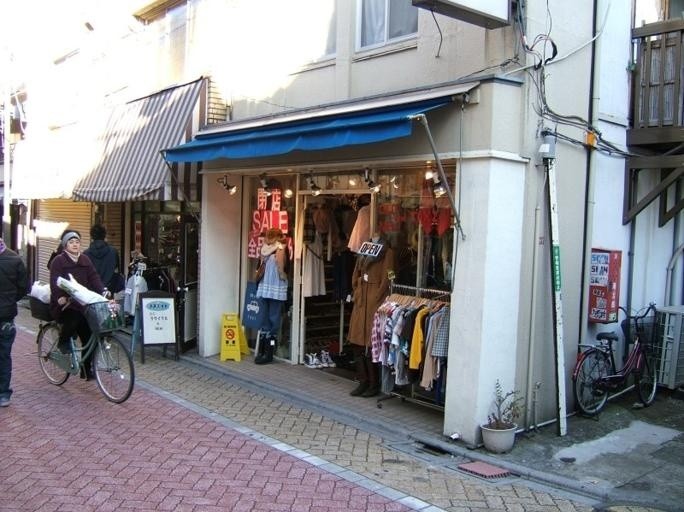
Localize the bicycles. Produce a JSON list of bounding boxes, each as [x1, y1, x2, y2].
[[570, 299, 667, 423], [27, 287, 137, 406]]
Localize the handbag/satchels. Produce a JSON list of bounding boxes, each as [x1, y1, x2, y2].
[[113, 272, 125, 292], [255, 263, 264, 281]]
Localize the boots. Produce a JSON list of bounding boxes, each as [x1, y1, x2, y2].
[[351, 358, 380, 397], [255, 336, 274, 364]]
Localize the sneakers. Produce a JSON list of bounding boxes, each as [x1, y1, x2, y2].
[[304, 350, 336, 368], [0, 394, 9, 407]]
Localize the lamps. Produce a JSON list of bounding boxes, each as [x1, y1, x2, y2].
[[216, 164, 458, 199]]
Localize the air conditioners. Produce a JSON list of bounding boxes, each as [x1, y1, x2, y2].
[[638, 303, 684, 396], [629, 35, 684, 133]]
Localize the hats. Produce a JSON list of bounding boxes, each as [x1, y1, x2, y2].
[[264, 228, 285, 244]]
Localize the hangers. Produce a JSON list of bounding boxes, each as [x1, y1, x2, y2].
[[374, 290, 447, 319], [126, 258, 169, 278]]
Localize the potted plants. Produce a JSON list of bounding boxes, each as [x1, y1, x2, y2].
[[480, 379, 524, 452]]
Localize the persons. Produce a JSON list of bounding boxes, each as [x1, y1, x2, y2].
[[83, 226, 119, 296], [254, 229, 289, 365], [49, 230, 117, 379], [0, 224, 26, 408], [46, 229, 80, 270], [349, 231, 394, 398]]
